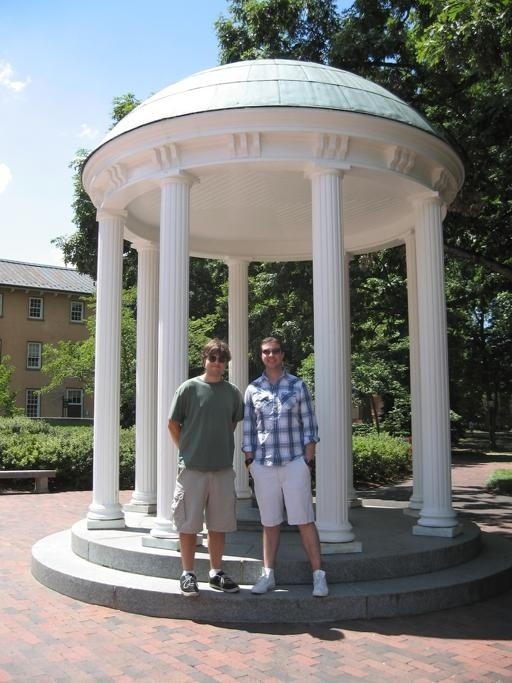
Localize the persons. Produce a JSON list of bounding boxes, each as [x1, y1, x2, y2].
[[167, 336, 246, 597], [241, 338, 329, 596]]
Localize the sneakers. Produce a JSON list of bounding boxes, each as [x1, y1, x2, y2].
[[209, 571, 239, 593], [251, 567, 275, 595], [312, 570, 328, 597], [180, 573, 200, 596]]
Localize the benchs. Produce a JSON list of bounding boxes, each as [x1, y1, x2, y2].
[[0, 470, 56, 493]]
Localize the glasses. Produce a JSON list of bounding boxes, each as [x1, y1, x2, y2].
[[205, 356, 229, 362], [262, 349, 282, 355]]
